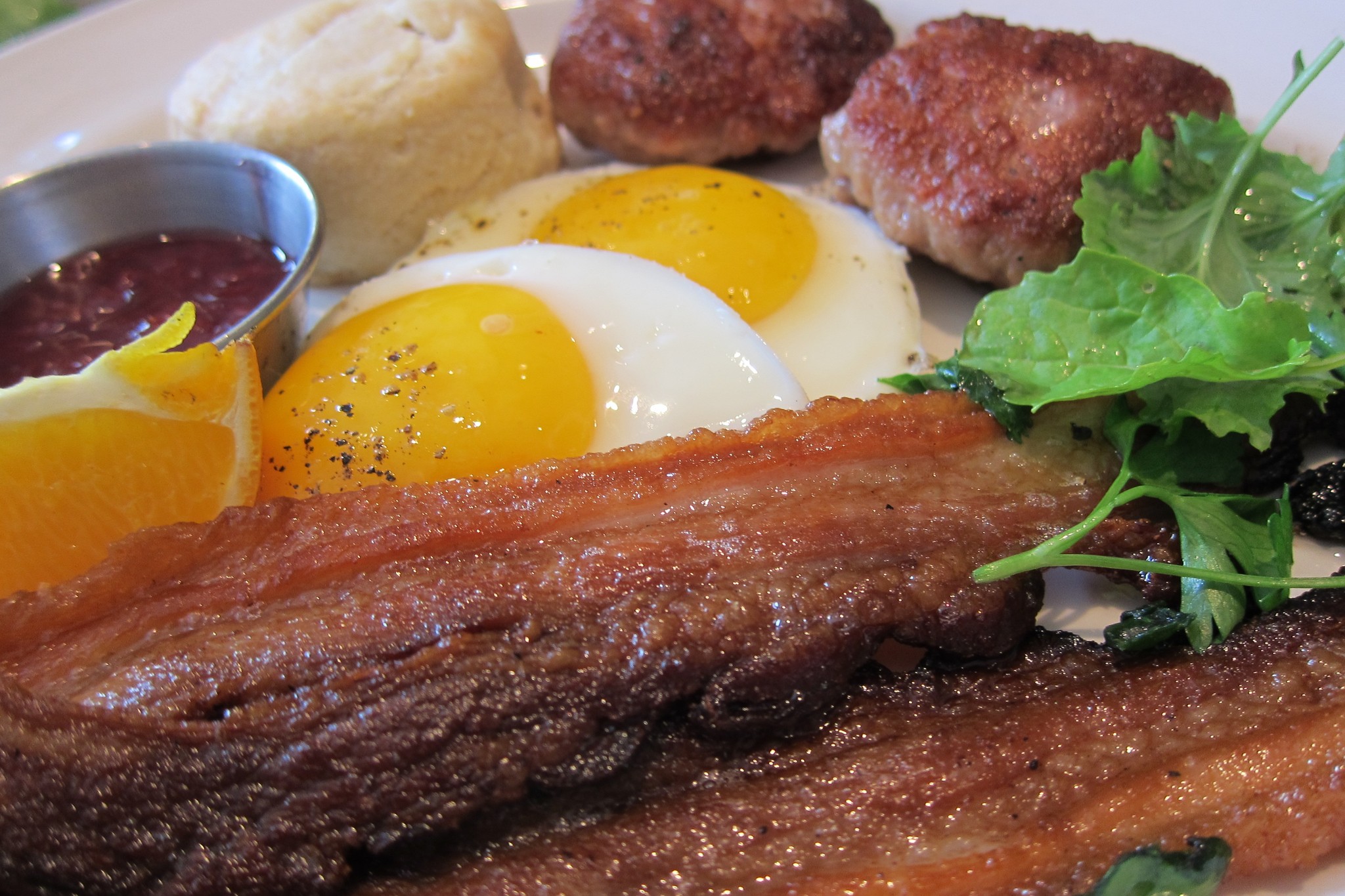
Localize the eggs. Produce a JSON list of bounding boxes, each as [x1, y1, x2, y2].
[[384, 159, 928, 405], [265, 247, 808, 502]]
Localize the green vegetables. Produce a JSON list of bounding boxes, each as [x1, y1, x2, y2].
[[872, 34, 1344, 650]]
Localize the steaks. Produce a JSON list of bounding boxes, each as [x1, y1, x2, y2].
[[1, 386, 1345, 896], [548, 0, 895, 166], [820, 12, 1237, 286]]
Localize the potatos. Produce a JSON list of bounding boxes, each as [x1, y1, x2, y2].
[[164, 1, 567, 287]]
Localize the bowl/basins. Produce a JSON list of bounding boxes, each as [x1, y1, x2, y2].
[[0, 137, 325, 395]]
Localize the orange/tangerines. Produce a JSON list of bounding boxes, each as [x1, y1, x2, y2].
[[1, 303, 263, 599]]
